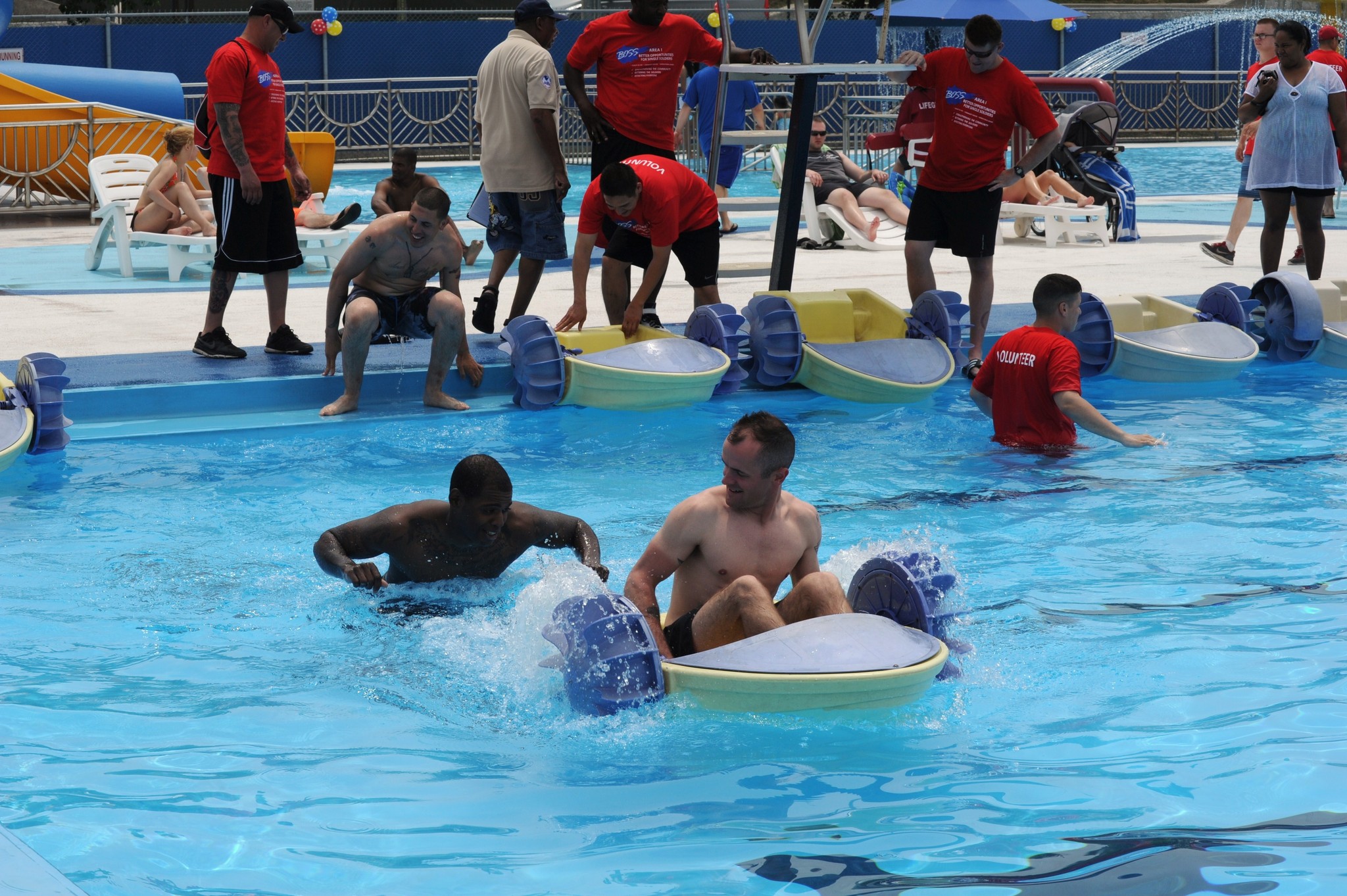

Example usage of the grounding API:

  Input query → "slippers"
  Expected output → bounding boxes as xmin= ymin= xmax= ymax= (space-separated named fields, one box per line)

xmin=330 ymin=203 xmax=362 ymax=231
xmin=719 ymin=223 xmax=738 ymax=237
xmin=796 ymin=237 xmax=844 ymax=250
xmin=962 ymin=359 xmax=984 ymax=380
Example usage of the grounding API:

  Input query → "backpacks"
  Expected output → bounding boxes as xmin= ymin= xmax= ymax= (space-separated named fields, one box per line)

xmin=194 ymin=40 xmax=250 ymax=160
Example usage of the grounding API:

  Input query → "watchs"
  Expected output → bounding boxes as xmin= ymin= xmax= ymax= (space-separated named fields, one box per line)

xmin=1250 ymin=98 xmax=1262 ymax=108
xmin=1014 ymin=165 xmax=1025 ymax=179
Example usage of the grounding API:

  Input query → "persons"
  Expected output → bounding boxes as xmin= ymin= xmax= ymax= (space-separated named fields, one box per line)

xmin=622 ymin=410 xmax=856 ymax=662
xmin=313 ymin=453 xmax=610 ymax=594
xmin=129 ymin=0 xmax=1347 ymax=455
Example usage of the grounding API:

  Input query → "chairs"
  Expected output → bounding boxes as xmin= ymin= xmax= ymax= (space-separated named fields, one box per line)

xmin=85 ymin=154 xmax=217 ymax=282
xmin=295 ymin=192 xmax=348 ymax=268
xmin=770 ymin=144 xmax=907 ymax=251
xmin=995 ymin=172 xmax=1109 ymax=248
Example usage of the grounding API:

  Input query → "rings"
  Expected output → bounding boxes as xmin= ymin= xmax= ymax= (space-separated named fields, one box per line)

xmin=883 ymin=174 xmax=886 ymax=175
xmin=994 ymin=180 xmax=996 ymax=184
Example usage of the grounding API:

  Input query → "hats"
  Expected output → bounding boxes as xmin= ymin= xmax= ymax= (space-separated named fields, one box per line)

xmin=249 ymin=0 xmax=305 ymax=34
xmin=1318 ymin=26 xmax=1343 ymax=40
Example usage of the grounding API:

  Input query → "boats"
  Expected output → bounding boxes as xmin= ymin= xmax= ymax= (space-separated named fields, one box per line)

xmin=1245 ymin=270 xmax=1347 ymax=373
xmin=534 ymin=541 xmax=971 ymax=717
xmin=1055 ymin=281 xmax=1267 ymax=384
xmin=739 ymin=286 xmax=972 ymax=407
xmin=0 ymin=352 xmax=74 ymax=474
xmin=498 ymin=303 xmax=753 ymax=414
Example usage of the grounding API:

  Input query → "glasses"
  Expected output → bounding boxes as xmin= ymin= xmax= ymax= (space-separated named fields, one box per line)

xmin=272 ymin=18 xmax=288 ymax=35
xmin=1251 ymin=33 xmax=1274 ymax=39
xmin=963 ymin=39 xmax=995 ymax=59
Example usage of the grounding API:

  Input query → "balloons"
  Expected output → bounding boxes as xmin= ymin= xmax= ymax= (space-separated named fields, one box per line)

xmin=1064 ymin=18 xmax=1076 ymax=21
xmin=713 ymin=1 xmax=729 ymax=14
xmin=728 ymin=13 xmax=734 ymax=25
xmin=707 ymin=13 xmax=721 ymax=27
xmin=1065 ymin=23 xmax=1078 ymax=32
xmin=1051 ymin=18 xmax=1065 ymax=30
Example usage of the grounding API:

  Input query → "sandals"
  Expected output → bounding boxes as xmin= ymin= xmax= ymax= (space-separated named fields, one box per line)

xmin=500 ymin=319 xmax=512 ymax=340
xmin=472 ymin=286 xmax=499 ymax=334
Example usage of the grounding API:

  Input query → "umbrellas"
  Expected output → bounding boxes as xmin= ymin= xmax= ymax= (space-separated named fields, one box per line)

xmin=868 ymin=0 xmax=1089 ymax=29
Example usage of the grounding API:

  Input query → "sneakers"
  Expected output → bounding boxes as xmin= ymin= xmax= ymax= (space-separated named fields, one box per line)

xmin=1287 ymin=245 xmax=1306 ymax=265
xmin=1199 ymin=241 xmax=1236 ymax=265
xmin=640 ymin=302 xmax=671 ymax=334
xmin=264 ymin=324 xmax=314 ymax=354
xmin=192 ymin=325 xmax=248 ymax=359
